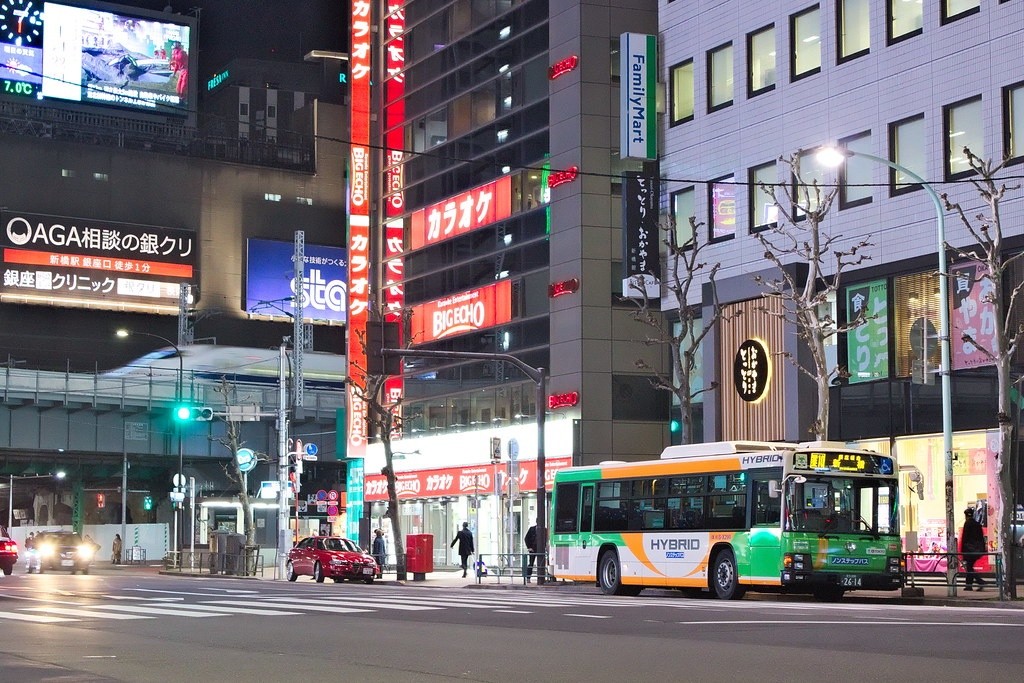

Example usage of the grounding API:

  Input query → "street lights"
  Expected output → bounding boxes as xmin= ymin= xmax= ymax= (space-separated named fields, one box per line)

xmin=8 ymin=472 xmax=66 ymax=540
xmin=117 ymin=330 xmax=183 ymax=567
xmin=816 ymin=144 xmax=958 ymax=598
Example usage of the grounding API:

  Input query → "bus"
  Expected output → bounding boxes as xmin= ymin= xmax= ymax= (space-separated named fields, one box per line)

xmin=550 ymin=441 xmax=924 ymax=602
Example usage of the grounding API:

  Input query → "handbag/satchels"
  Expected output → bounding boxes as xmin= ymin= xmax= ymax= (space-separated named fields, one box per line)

xmin=91 ymin=543 xmax=101 ymax=553
xmin=376 ymin=564 xmax=380 ymax=574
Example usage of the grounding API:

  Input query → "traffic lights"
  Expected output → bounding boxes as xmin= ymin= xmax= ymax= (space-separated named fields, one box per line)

xmin=144 ymin=496 xmax=152 ymax=510
xmin=174 ymin=406 xmax=213 ymax=422
xmin=98 ymin=494 xmax=105 ymax=508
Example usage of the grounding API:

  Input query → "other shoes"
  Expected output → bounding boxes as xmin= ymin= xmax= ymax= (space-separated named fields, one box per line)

xmin=977 ymin=580 xmax=986 ymax=591
xmin=964 ymin=587 xmax=974 ymax=591
xmin=527 ymin=579 xmax=531 ymax=583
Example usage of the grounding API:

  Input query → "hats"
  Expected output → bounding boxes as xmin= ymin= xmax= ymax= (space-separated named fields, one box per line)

xmin=964 ymin=508 xmax=975 ymax=517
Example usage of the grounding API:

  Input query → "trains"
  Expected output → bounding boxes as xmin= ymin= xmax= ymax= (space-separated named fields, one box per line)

xmin=103 ymin=344 xmax=346 ymax=388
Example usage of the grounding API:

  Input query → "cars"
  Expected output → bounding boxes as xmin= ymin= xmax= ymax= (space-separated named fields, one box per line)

xmin=26 ymin=530 xmax=89 ymax=575
xmin=284 ymin=536 xmax=378 ymax=584
xmin=0 ymin=525 xmax=18 ymax=575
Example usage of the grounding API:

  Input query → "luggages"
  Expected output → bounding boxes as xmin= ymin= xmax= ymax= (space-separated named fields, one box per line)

xmin=470 ymin=554 xmax=488 ymax=578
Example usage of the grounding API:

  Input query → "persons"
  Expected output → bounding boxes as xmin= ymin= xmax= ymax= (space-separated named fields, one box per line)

xmin=372 ymin=529 xmax=387 ymax=579
xmin=960 ymin=508 xmax=986 ymax=590
xmin=450 ymin=522 xmax=475 ymax=578
xmin=25 ymin=530 xmax=40 ymax=547
xmin=152 ymin=41 xmax=189 ymax=98
xmin=112 ymin=534 xmax=122 ymax=564
xmin=525 ymin=518 xmax=547 ymax=583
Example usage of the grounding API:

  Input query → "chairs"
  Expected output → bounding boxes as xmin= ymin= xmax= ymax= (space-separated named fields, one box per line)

xmin=582 ymin=504 xmax=703 ymax=529
